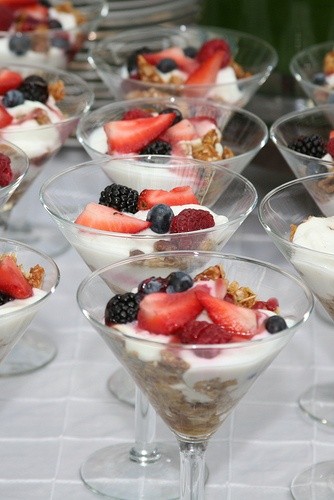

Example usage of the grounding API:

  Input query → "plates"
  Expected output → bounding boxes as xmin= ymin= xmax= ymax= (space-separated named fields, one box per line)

xmin=60 ymin=1 xmax=201 ymax=150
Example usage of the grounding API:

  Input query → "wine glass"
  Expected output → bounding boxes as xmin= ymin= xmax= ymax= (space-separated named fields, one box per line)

xmin=76 ymin=97 xmax=268 ymax=409
xmin=75 ymin=250 xmax=315 ymax=499
xmin=0 ymin=0 xmax=108 ymax=258
xmin=255 ymin=172 xmax=334 ymax=500
xmin=268 ymin=103 xmax=333 ymax=428
xmin=39 ymin=154 xmax=259 ymax=500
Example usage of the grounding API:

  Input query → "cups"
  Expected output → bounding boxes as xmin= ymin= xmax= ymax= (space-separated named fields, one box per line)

xmin=0 ymin=237 xmax=61 ymax=370
xmin=0 ymin=61 xmax=95 ymax=223
xmin=88 ymin=23 xmax=279 ymax=111
xmin=286 ymin=34 xmax=334 ymax=106
xmin=0 ymin=135 xmax=31 ymax=216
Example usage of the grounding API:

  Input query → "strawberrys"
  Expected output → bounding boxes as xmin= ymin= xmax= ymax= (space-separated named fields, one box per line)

xmin=139 ymin=284 xmax=268 ymax=340
xmin=327 ymin=131 xmax=334 ymax=158
xmin=0 ymin=6 xmax=223 ymax=300
